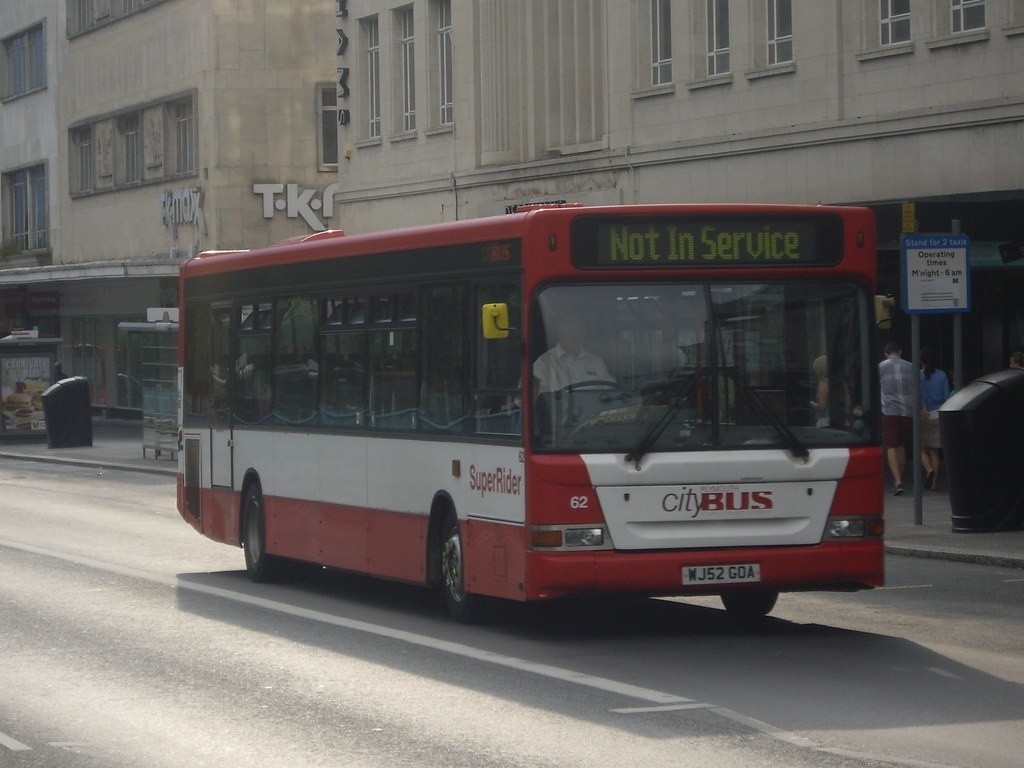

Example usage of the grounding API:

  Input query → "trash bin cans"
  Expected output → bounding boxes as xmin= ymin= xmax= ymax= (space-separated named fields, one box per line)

xmin=937 ymin=369 xmax=1024 ymax=534
xmin=40 ymin=376 xmax=92 ymax=449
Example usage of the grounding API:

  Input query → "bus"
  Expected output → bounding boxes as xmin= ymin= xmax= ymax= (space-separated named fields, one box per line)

xmin=174 ymin=202 xmax=897 ymax=623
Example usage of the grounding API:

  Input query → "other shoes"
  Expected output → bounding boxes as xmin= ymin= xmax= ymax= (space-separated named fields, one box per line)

xmin=926 ymin=471 xmax=935 ymax=488
xmin=930 ymin=484 xmax=938 ymax=490
xmin=892 ymin=484 xmax=904 ymax=496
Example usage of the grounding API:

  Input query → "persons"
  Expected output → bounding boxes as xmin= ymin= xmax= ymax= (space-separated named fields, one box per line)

xmin=809 ymin=355 xmax=850 ymax=430
xmin=1010 ymin=353 xmax=1024 ymax=369
xmin=513 ymin=317 xmax=616 ymax=407
xmin=919 ymin=348 xmax=949 ymax=491
xmin=54 ymin=362 xmax=68 ymax=384
xmin=877 ymin=342 xmax=912 ymax=495
